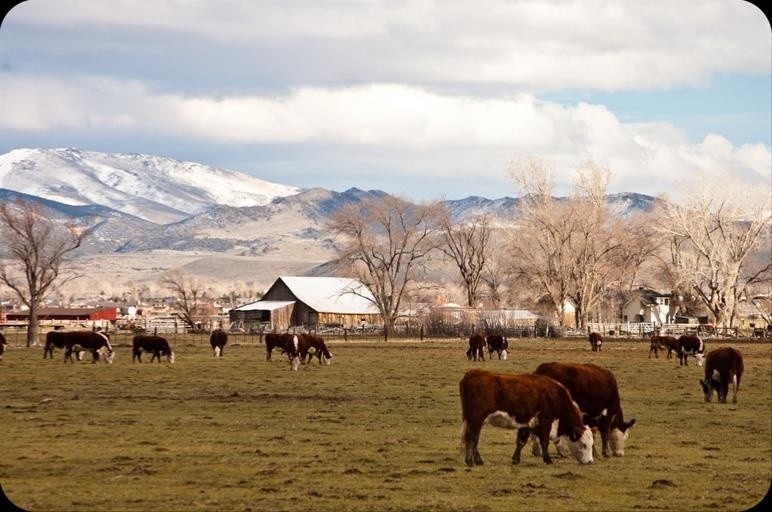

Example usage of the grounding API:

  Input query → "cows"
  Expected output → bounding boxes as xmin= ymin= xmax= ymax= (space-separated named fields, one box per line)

xmin=648 ymin=336 xmax=682 ymax=359
xmin=42 ymin=331 xmax=115 ymax=365
xmin=589 ymin=332 xmax=603 ymax=352
xmin=0 ymin=332 xmax=10 ymax=362
xmin=456 ymin=368 xmax=597 ymax=464
xmin=210 ymin=329 xmax=228 ymax=357
xmin=531 ymin=361 xmax=636 ymax=458
xmin=265 ymin=333 xmax=333 ymax=371
xmin=699 ymin=347 xmax=744 ymax=411
xmin=465 ymin=335 xmax=487 ymax=362
xmin=132 ymin=335 xmax=176 ymax=366
xmin=676 ymin=336 xmax=707 ymax=367
xmin=486 ymin=335 xmax=509 ymax=362
